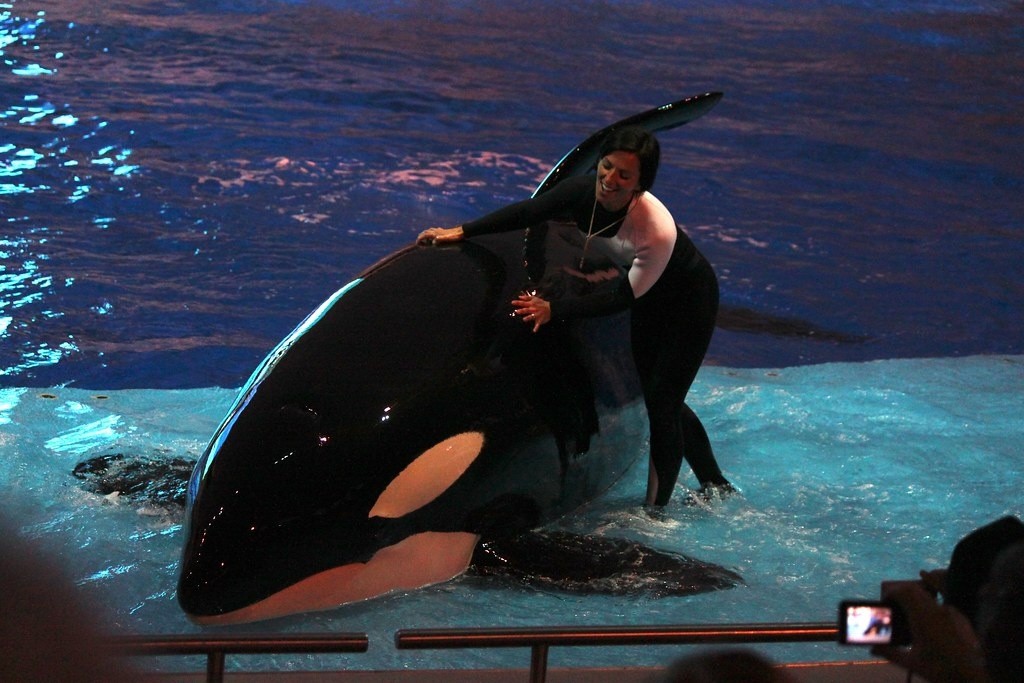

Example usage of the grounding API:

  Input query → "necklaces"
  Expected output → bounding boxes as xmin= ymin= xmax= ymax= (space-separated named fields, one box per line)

xmin=576 ymin=191 xmax=640 ymax=268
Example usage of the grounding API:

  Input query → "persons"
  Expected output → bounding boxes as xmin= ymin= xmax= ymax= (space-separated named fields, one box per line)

xmin=658 ymin=514 xmax=1023 ymax=683
xmin=416 ymin=126 xmax=736 ymax=521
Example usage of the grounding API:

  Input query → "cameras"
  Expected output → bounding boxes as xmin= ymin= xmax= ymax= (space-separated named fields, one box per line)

xmin=837 ymin=601 xmax=914 ymax=647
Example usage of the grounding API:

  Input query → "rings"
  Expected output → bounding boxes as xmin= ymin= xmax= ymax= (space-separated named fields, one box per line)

xmin=531 ymin=314 xmax=535 ymax=319
